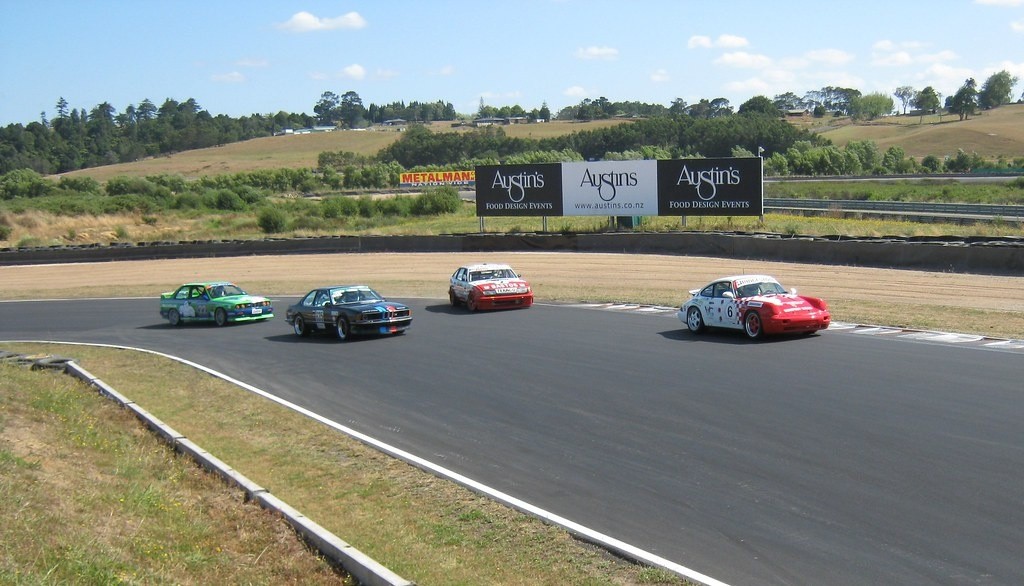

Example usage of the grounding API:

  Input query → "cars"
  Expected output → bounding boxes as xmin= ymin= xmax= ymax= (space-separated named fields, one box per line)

xmin=284 ymin=285 xmax=412 ymax=343
xmin=449 ymin=263 xmax=533 ymax=312
xmin=160 ymin=281 xmax=274 ymax=326
xmin=678 ymin=274 xmax=832 ymax=343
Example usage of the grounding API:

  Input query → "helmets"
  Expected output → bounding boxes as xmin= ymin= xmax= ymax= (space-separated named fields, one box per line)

xmin=216 ymin=287 xmax=225 ymax=294
